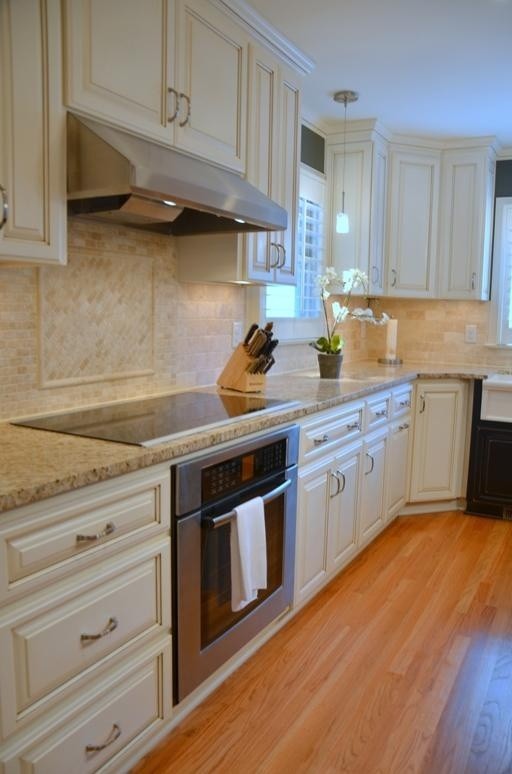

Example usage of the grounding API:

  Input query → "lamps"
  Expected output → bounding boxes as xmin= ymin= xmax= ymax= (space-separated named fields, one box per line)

xmin=332 ymin=90 xmax=358 ymax=235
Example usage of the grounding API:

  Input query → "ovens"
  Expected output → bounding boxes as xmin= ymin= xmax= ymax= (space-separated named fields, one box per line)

xmin=170 ymin=424 xmax=301 ymax=709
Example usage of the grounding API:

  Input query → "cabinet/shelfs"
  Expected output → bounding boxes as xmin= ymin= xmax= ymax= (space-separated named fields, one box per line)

xmin=66 ymin=1 xmax=249 ymax=180
xmin=385 ymin=134 xmax=437 ymax=300
xmin=317 ymin=118 xmax=385 ymax=298
xmin=309 ymin=266 xmax=390 ymax=356
xmin=0 ymin=462 xmax=175 ymax=774
xmin=174 ymin=0 xmax=315 ymax=287
xmin=437 ymin=136 xmax=497 ymax=302
xmin=1 ymin=0 xmax=67 ymax=268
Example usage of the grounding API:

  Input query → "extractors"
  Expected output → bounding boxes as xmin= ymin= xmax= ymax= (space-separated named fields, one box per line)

xmin=66 ymin=112 xmax=288 ymax=237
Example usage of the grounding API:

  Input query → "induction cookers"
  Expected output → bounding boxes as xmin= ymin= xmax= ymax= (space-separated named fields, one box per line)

xmin=10 ymin=391 xmax=303 ymax=450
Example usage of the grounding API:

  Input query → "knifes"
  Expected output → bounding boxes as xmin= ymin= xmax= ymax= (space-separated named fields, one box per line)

xmin=242 ymin=320 xmax=279 ymax=375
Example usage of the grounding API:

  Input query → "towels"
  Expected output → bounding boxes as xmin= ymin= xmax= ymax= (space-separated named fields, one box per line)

xmin=229 ymin=496 xmax=268 ymax=612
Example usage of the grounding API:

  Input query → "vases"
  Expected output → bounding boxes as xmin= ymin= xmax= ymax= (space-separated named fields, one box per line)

xmin=317 ymin=354 xmax=343 ymax=380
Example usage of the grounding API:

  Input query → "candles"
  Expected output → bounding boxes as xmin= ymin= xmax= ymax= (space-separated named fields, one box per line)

xmin=385 ymin=315 xmax=397 ymax=360
